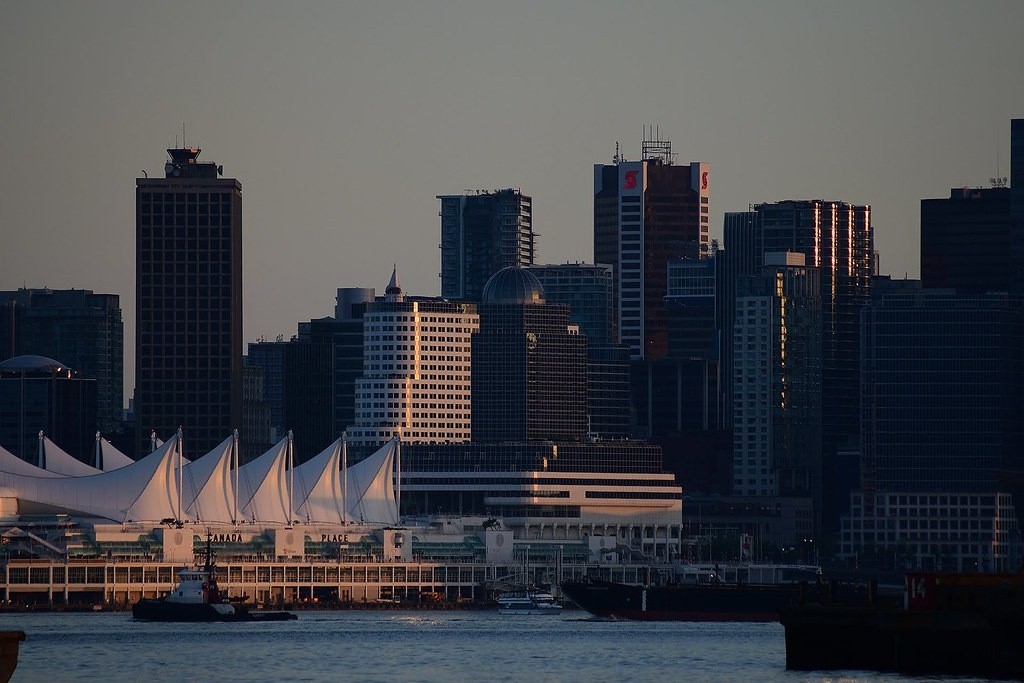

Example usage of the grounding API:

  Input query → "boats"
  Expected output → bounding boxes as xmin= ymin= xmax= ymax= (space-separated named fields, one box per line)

xmin=498 ymin=587 xmax=562 ymax=615
xmin=132 ymin=528 xmax=298 ymax=622
xmin=561 ymin=571 xmax=879 ymax=622
